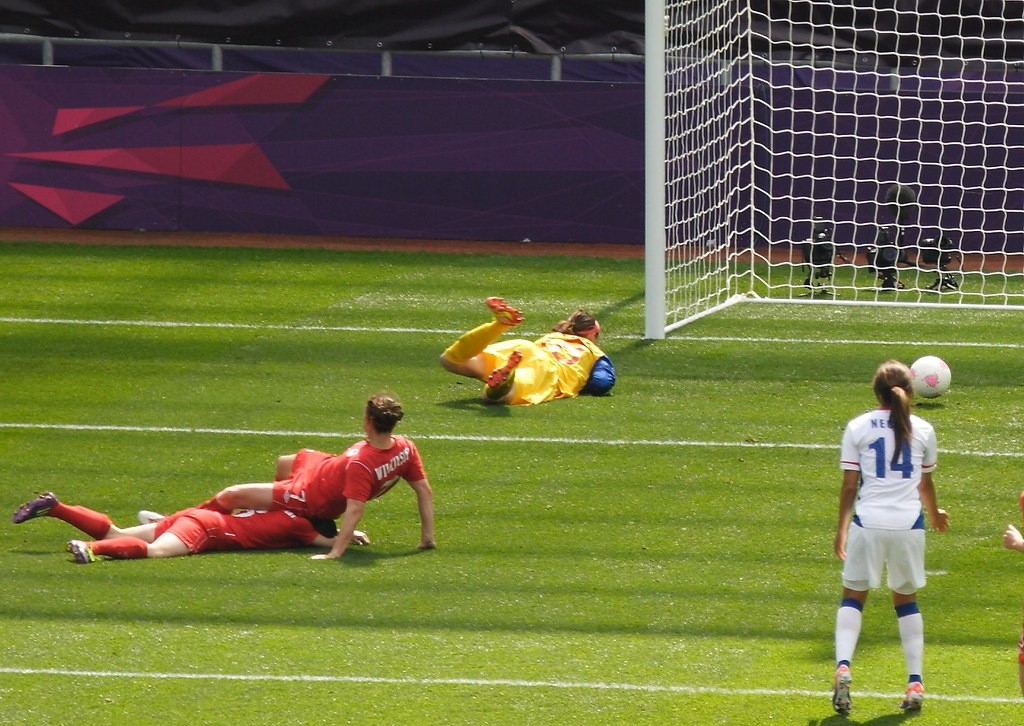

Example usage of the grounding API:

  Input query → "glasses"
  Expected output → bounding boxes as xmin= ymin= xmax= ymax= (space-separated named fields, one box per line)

xmin=594 ymin=333 xmax=600 ymax=338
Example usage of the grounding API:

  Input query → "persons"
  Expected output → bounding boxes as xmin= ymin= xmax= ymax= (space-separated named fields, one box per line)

xmin=15 ymin=490 xmax=371 ymax=566
xmin=440 ymin=295 xmax=618 ymax=406
xmin=138 ymin=394 xmax=437 ymax=560
xmin=1002 ymin=491 xmax=1024 ymax=699
xmin=830 ymin=358 xmax=952 ymax=713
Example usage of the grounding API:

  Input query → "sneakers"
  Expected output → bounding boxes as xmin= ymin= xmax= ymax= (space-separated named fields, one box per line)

xmin=13 ymin=491 xmax=57 ymax=524
xmin=486 ymin=297 xmax=524 ymax=326
xmin=900 ymin=682 xmax=923 ymax=711
xmin=67 ymin=540 xmax=113 ymax=564
xmin=487 ymin=351 xmax=523 ymax=388
xmin=829 ymin=665 xmax=851 ymax=715
xmin=137 ymin=510 xmax=165 ymax=524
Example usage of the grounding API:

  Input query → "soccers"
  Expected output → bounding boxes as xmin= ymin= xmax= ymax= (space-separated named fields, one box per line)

xmin=908 ymin=356 xmax=952 ymax=400
